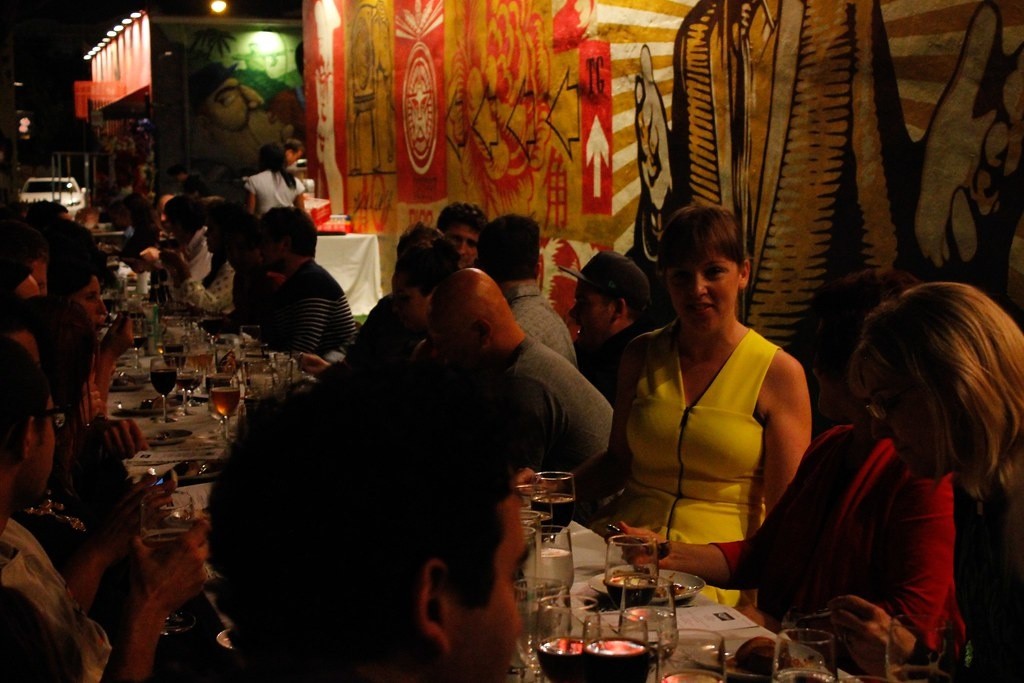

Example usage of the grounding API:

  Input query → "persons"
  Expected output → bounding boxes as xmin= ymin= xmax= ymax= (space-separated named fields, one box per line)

xmin=607 ymin=264 xmax=964 ymax=682
xmin=510 ymin=205 xmax=813 ymax=631
xmin=850 ymin=281 xmax=1024 ymax=683
xmin=0 ymin=140 xmax=668 ymax=683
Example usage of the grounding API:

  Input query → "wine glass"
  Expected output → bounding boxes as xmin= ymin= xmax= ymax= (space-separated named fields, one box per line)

xmin=132 ymin=316 xmax=304 ymax=447
xmin=139 ymin=488 xmax=197 ymax=636
xmin=514 ymin=471 xmax=725 ymax=683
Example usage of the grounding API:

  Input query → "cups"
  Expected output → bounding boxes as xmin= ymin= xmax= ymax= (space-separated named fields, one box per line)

xmin=773 ymin=626 xmax=841 ymax=683
xmin=883 ymin=614 xmax=961 ymax=682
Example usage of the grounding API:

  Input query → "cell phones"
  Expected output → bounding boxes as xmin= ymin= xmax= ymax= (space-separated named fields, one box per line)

xmin=154 ymin=473 xmax=172 ymax=485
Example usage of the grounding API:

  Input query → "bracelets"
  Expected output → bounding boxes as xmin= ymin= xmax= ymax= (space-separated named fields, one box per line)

xmin=151 ymin=260 xmax=161 ymax=266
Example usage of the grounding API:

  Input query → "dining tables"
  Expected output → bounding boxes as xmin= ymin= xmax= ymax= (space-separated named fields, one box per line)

xmin=101 ymin=236 xmax=865 ymax=683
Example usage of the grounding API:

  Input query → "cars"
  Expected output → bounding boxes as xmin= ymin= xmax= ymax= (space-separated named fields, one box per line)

xmin=18 ymin=178 xmax=86 ymax=218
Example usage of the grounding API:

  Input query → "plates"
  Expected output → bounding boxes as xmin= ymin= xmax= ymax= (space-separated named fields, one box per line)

xmin=582 ymin=566 xmax=703 ymax=601
xmin=694 ymin=636 xmax=825 ymax=682
xmin=216 ymin=627 xmax=232 ymax=649
xmin=110 ymin=353 xmax=280 ymax=482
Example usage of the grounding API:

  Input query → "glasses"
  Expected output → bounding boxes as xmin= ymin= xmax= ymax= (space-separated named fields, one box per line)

xmin=865 ymin=380 xmax=922 ymax=419
xmin=26 ymin=401 xmax=71 ymax=428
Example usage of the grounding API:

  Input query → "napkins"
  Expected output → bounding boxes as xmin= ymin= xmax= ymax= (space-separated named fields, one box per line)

xmin=550 ymin=521 xmax=627 ymax=570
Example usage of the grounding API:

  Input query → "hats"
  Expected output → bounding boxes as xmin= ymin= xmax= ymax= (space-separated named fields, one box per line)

xmin=559 ymin=249 xmax=648 ymax=307
